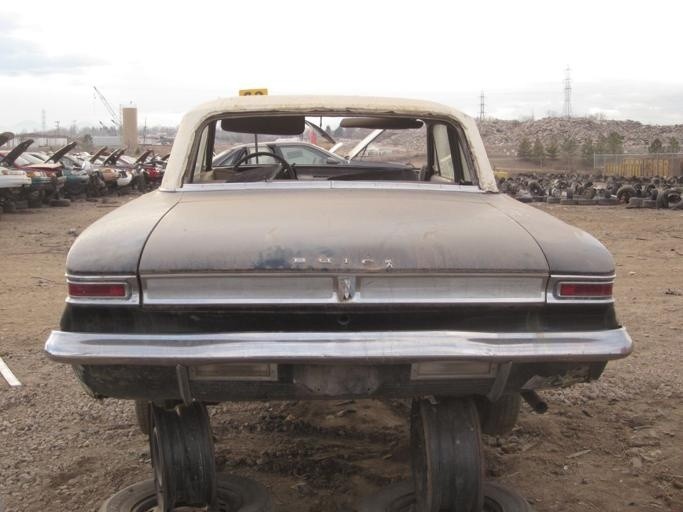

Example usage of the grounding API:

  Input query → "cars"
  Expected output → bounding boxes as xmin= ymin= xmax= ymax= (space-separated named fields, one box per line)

xmin=44 ymin=92 xmax=635 ymax=510
xmin=1 ymin=128 xmax=170 ymax=209
